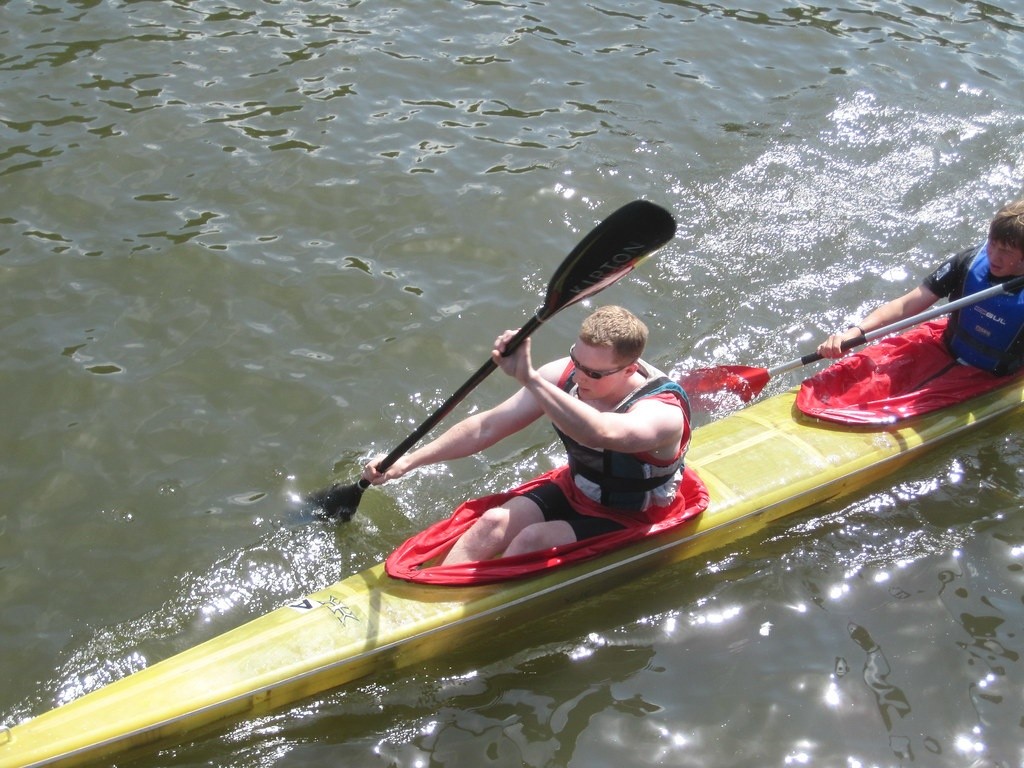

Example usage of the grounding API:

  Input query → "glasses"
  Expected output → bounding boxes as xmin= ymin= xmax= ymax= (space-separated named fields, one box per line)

xmin=569 ymin=344 xmax=635 ymax=379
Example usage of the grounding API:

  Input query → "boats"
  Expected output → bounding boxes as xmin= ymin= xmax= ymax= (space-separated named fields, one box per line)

xmin=0 ymin=310 xmax=1022 ymax=768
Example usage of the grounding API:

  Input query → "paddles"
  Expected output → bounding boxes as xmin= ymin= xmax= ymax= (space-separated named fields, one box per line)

xmin=301 ymin=196 xmax=691 ymax=533
xmin=662 ymin=266 xmax=1024 ymax=423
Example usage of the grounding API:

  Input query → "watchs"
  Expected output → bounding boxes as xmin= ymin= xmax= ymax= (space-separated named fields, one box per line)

xmin=848 ymin=323 xmax=865 ymax=336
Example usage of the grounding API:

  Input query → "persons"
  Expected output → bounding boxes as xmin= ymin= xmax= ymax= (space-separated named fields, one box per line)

xmin=817 ymin=200 xmax=1024 ymax=377
xmin=362 ymin=305 xmax=692 ymax=565
